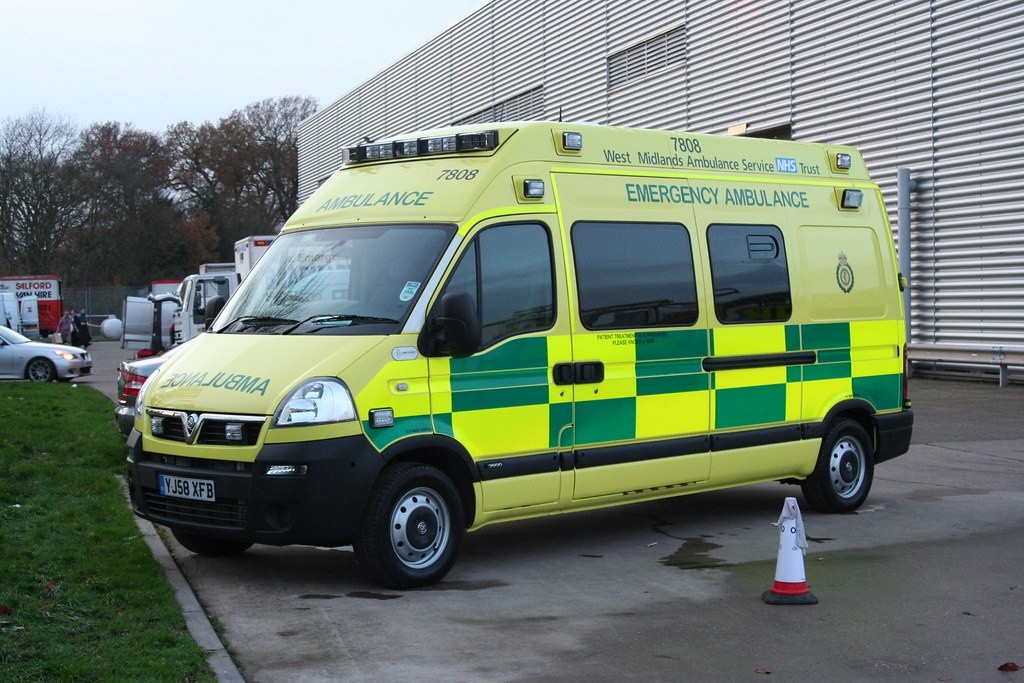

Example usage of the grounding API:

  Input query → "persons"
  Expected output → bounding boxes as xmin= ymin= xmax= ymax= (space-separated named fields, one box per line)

xmin=68 ymin=309 xmax=93 ymax=350
xmin=56 ymin=310 xmax=79 ymax=346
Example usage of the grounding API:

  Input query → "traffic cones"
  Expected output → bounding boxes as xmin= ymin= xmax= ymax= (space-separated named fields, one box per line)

xmin=759 ymin=496 xmax=821 ymax=607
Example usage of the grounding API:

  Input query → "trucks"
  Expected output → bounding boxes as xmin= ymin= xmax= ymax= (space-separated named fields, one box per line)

xmin=170 ymin=234 xmax=350 ymax=348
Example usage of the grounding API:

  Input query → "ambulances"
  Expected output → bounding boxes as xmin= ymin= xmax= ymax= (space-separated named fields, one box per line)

xmin=124 ymin=120 xmax=918 ymax=589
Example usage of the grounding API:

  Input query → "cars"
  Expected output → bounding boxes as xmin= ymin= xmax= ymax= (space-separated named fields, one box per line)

xmin=0 ymin=325 xmax=94 ymax=385
xmin=112 ymin=336 xmax=198 ymax=439
